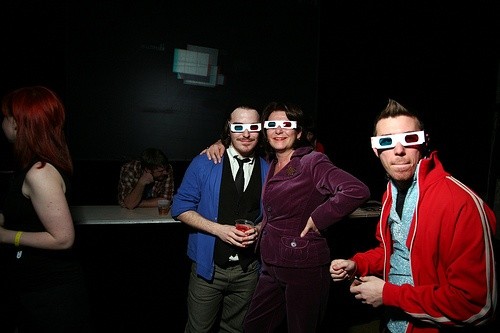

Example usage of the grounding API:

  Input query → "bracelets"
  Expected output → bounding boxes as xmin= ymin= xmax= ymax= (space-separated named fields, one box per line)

xmin=15 ymin=232 xmax=22 ymax=247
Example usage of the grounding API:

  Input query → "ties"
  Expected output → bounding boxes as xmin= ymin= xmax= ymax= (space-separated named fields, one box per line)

xmin=231 ymin=155 xmax=251 ymax=257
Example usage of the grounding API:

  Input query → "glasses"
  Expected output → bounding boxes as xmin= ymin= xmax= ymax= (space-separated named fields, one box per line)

xmin=264 ymin=121 xmax=297 ymax=129
xmin=370 ymin=131 xmax=425 ymax=149
xmin=228 ymin=121 xmax=261 ymax=132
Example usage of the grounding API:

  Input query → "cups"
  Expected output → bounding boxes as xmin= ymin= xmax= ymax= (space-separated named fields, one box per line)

xmin=157 ymin=199 xmax=170 ymax=216
xmin=235 ymin=219 xmax=253 ymax=242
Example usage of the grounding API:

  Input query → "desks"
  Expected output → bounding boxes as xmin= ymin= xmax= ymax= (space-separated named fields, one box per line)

xmin=69 ymin=206 xmax=193 ymax=224
xmin=347 ymin=202 xmax=382 ymax=218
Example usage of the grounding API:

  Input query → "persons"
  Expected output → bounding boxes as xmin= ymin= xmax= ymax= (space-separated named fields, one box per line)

xmin=330 ymin=99 xmax=500 ymax=333
xmin=118 ymin=148 xmax=175 ymax=209
xmin=198 ymin=102 xmax=371 ymax=333
xmin=171 ymin=101 xmax=271 ymax=333
xmin=0 ymin=86 xmax=94 ymax=333
